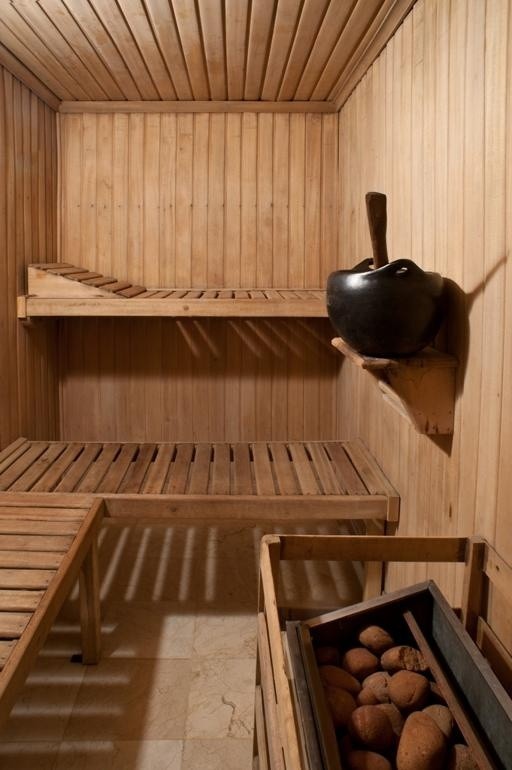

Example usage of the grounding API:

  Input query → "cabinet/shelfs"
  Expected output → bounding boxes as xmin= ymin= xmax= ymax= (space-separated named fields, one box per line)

xmin=2 ymin=491 xmax=106 ymax=722
xmin=2 ymin=434 xmax=401 ymax=601
